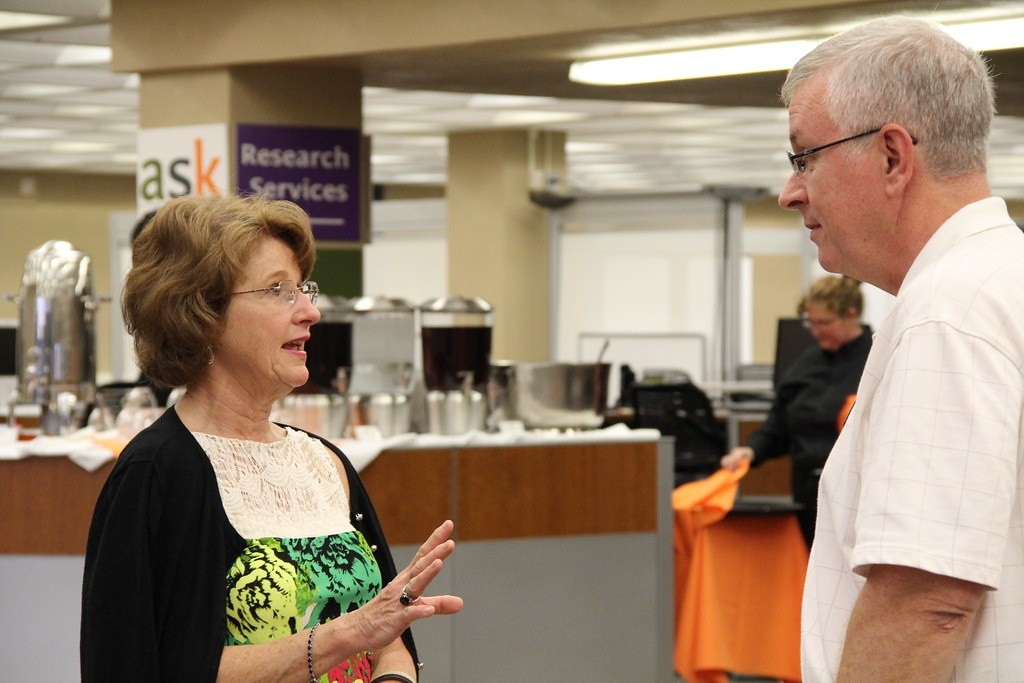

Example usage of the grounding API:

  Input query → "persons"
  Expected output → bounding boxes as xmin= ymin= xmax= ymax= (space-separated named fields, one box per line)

xmin=80 ymin=197 xmax=464 ymax=683
xmin=721 ymin=274 xmax=875 ymax=554
xmin=778 ymin=17 xmax=1024 ymax=683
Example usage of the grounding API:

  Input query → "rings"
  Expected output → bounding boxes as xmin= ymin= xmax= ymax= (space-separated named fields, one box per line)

xmin=399 ymin=584 xmax=421 ymax=606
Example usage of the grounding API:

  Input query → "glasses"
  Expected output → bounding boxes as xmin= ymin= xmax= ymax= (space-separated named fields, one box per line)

xmin=802 ymin=317 xmax=838 ymax=329
xmin=210 ymin=279 xmax=319 ymax=307
xmin=786 ymin=127 xmax=921 ymax=174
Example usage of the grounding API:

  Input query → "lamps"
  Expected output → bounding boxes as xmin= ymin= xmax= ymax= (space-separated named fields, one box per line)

xmin=568 ymin=0 xmax=1024 ymax=86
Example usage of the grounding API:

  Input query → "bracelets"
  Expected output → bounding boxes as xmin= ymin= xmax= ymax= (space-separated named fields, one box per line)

xmin=370 ymin=670 xmax=414 ymax=683
xmin=307 ymin=622 xmax=320 ymax=683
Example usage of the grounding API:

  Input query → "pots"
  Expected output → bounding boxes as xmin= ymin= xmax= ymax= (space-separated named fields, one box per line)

xmin=489 ymin=359 xmax=611 ymax=428
